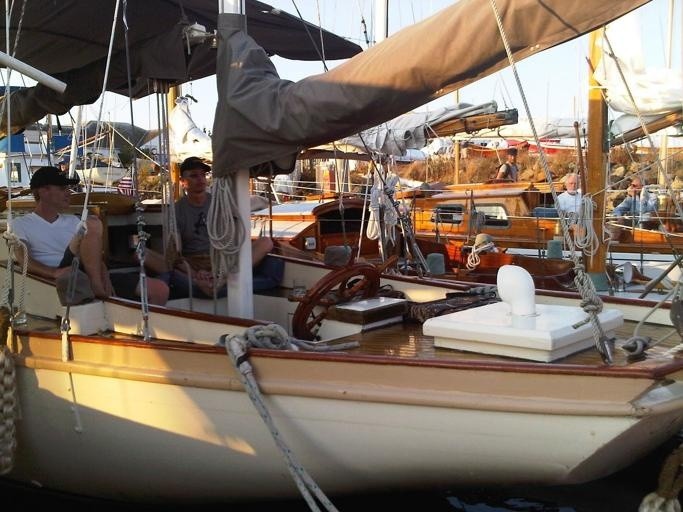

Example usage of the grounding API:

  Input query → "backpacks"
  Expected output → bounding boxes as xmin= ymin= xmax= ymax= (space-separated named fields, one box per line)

xmin=488 ymin=162 xmax=510 ymax=181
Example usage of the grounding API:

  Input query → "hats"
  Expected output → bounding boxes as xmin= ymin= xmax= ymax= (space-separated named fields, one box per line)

xmin=507 ymin=149 xmax=518 ymax=156
xmin=178 ymin=157 xmax=212 ymax=176
xmin=28 ymin=165 xmax=81 ymax=187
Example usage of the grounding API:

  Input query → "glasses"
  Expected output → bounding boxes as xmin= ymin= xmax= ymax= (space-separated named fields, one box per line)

xmin=630 ymin=183 xmax=642 ymax=188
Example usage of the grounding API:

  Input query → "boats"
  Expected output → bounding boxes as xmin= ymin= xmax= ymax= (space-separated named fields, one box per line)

xmin=507 ymin=139 xmax=575 ymax=155
xmin=463 ymin=139 xmax=530 ymax=161
xmin=1 ymin=105 xmax=164 ymax=197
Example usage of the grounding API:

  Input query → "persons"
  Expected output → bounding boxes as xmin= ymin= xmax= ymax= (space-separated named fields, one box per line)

xmin=612 ymin=177 xmax=658 ymax=224
xmin=135 ymin=157 xmax=273 ymax=299
xmin=495 ymin=148 xmax=518 ymax=183
xmin=11 ymin=166 xmax=168 ymax=306
xmin=556 ymin=173 xmax=581 ymax=214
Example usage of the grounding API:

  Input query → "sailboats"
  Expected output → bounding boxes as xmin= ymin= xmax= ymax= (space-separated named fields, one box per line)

xmin=393 ymin=87 xmax=682 ymax=256
xmin=0 ymin=0 xmax=682 ymax=497
xmin=168 ymin=2 xmax=683 ymax=301
